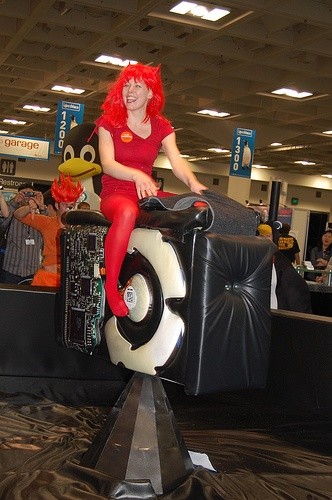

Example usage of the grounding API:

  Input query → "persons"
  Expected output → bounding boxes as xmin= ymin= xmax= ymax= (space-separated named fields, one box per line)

xmin=94 ymin=63 xmax=210 ymax=317
xmin=255 ymin=220 xmax=332 ymax=314
xmin=0 ymin=172 xmax=91 ymax=287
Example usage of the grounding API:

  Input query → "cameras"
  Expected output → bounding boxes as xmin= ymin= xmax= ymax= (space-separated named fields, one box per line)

xmin=24 ymin=192 xmax=36 ymax=198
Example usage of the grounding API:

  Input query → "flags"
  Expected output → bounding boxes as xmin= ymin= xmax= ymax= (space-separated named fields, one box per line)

xmin=54 ymin=100 xmax=84 ymax=156
xmin=229 ymin=128 xmax=256 ymax=179
